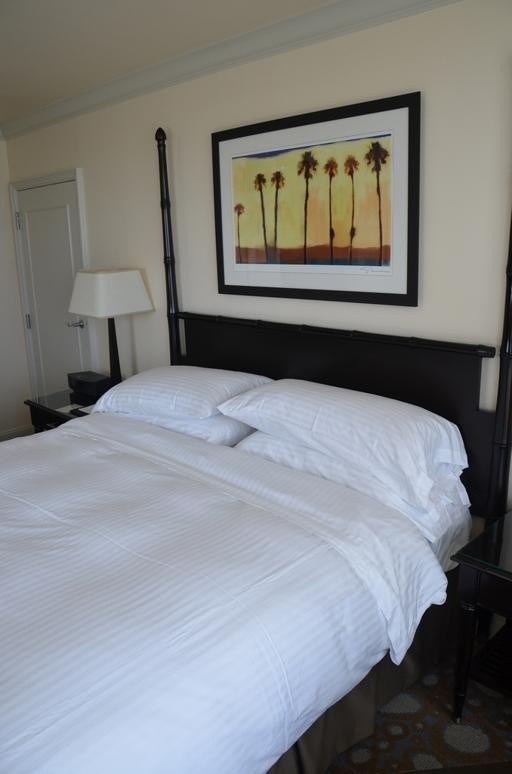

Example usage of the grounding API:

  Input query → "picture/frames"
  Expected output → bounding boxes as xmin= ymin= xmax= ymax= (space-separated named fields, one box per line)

xmin=209 ymin=89 xmax=420 ymax=307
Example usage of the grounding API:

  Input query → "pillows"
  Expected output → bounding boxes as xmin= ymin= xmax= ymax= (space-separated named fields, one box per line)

xmin=219 ymin=378 xmax=468 ymax=503
xmin=90 ymin=365 xmax=274 ymax=415
xmin=134 ymin=410 xmax=250 ymax=448
xmin=234 ymin=429 xmax=468 ymax=543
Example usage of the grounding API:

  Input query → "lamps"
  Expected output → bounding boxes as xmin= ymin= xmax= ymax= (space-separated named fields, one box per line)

xmin=66 ymin=268 xmax=155 ymax=387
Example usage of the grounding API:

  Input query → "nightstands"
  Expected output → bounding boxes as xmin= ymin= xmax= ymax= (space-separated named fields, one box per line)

xmin=22 ymin=389 xmax=97 ymax=434
xmin=451 ymin=507 xmax=511 ymax=724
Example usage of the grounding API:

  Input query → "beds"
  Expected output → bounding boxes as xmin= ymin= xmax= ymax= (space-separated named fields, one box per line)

xmin=0 ymin=311 xmax=508 ymax=774
